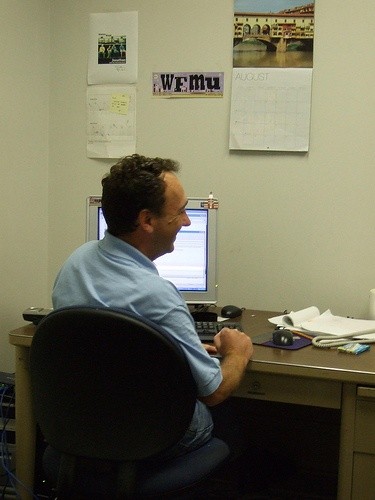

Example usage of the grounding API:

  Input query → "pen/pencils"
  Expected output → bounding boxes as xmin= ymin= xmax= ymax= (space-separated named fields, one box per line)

xmin=291 ymin=330 xmax=313 ymax=340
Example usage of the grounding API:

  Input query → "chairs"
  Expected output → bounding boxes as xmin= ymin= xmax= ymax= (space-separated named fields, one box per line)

xmin=27 ymin=304 xmax=230 ymax=500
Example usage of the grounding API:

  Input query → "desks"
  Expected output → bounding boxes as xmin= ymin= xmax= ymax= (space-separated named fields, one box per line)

xmin=8 ymin=306 xmax=375 ymax=500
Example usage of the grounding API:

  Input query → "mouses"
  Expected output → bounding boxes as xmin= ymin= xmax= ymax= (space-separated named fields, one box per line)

xmin=221 ymin=305 xmax=242 ymax=318
xmin=274 ymin=330 xmax=293 ymax=346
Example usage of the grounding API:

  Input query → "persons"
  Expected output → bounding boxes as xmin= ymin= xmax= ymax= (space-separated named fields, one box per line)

xmin=99 ymin=43 xmax=126 ymax=58
xmin=52 ymin=154 xmax=271 ymax=500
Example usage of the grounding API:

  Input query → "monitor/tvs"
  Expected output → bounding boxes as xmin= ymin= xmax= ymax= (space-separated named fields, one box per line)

xmin=85 ymin=195 xmax=217 ymax=302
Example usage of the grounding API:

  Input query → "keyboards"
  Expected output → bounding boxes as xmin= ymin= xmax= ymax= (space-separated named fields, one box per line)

xmin=193 ymin=321 xmax=241 ymax=342
